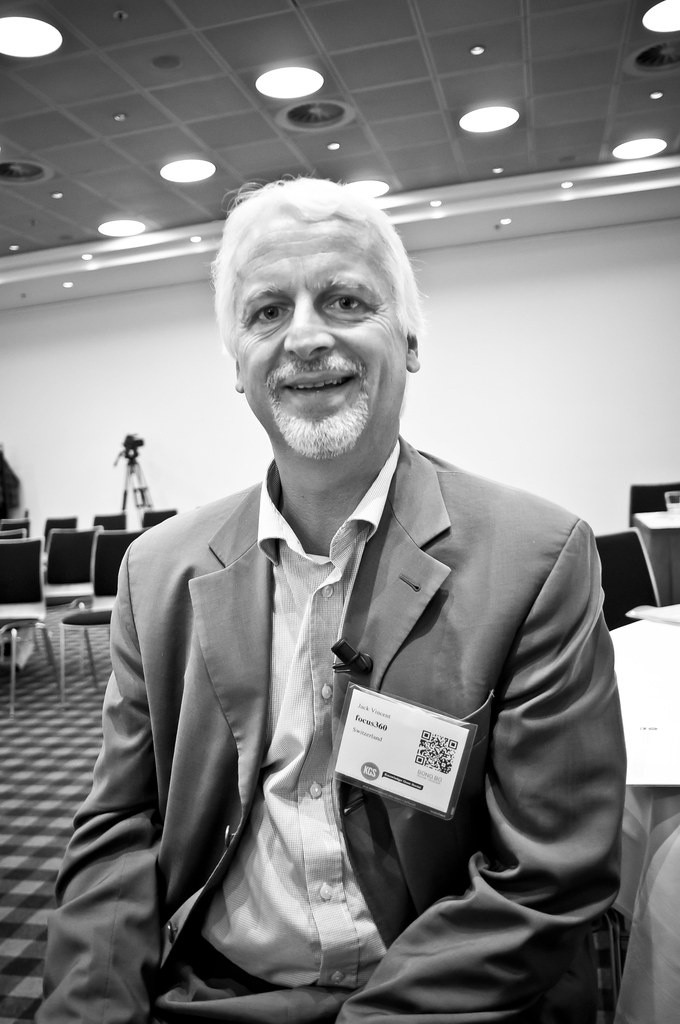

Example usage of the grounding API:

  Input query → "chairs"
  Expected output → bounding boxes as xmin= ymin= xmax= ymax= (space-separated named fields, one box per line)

xmin=594 ymin=529 xmax=658 ymax=630
xmin=0 ymin=509 xmax=178 ymax=719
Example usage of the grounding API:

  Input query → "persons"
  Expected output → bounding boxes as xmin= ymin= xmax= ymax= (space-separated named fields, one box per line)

xmin=38 ymin=179 xmax=626 ymax=1024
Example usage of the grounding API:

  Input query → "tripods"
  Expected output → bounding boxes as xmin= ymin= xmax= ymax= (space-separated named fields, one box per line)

xmin=114 ymin=448 xmax=156 ymax=527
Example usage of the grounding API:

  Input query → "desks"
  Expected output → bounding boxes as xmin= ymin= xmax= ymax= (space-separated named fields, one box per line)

xmin=610 ymin=603 xmax=680 ymax=787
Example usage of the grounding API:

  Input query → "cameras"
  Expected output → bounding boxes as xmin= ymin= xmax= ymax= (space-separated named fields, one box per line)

xmin=124 ymin=436 xmax=144 ymax=449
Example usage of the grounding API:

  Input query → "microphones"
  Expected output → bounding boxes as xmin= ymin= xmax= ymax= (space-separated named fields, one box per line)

xmin=331 ymin=639 xmax=374 ymax=675
